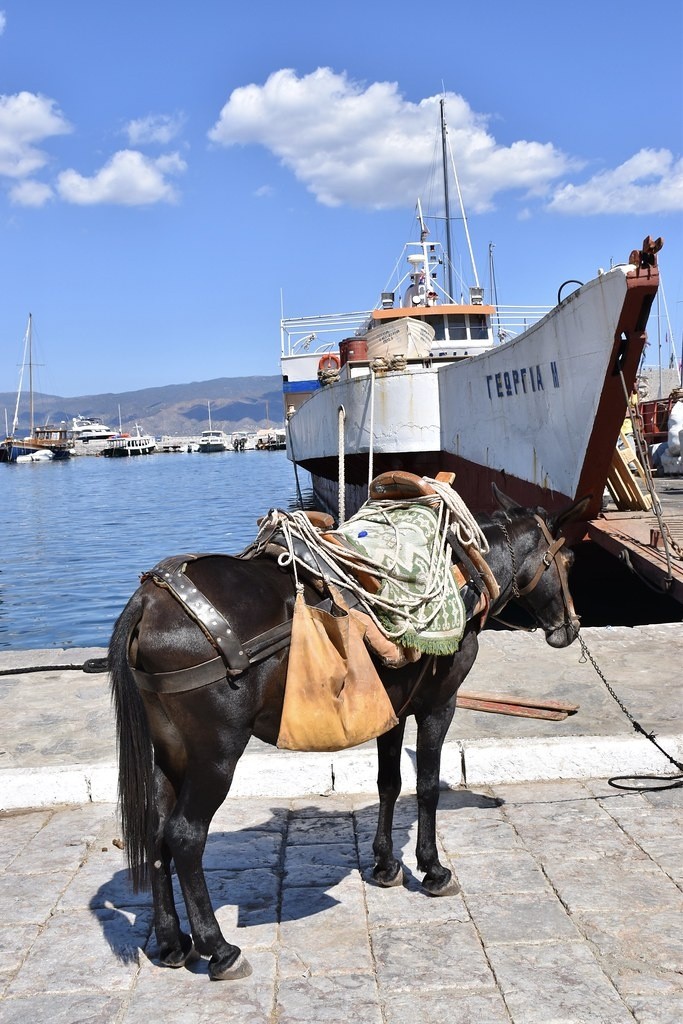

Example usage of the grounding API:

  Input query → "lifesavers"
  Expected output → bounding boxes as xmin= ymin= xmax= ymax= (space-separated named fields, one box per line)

xmin=240 ymin=439 xmax=244 ymax=446
xmin=318 ymin=354 xmax=340 ymax=371
xmin=234 ymin=439 xmax=238 ymax=447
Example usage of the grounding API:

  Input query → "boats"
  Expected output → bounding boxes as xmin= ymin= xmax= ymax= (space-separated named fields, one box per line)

xmin=279 ymin=82 xmax=667 ymax=551
xmin=54 ymin=398 xmax=287 ymax=457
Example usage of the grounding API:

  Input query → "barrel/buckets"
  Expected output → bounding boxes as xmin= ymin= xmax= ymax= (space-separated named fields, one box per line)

xmin=339 ymin=337 xmax=368 ymax=368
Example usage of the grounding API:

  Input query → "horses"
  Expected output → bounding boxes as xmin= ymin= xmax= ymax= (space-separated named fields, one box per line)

xmin=104 ymin=483 xmax=593 ymax=982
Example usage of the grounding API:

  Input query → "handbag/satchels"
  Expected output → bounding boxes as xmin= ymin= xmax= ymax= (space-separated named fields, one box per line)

xmin=276 ymin=583 xmax=400 ymax=753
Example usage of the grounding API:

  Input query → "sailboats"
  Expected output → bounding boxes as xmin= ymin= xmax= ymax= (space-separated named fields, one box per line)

xmin=0 ymin=312 xmax=76 ymax=464
